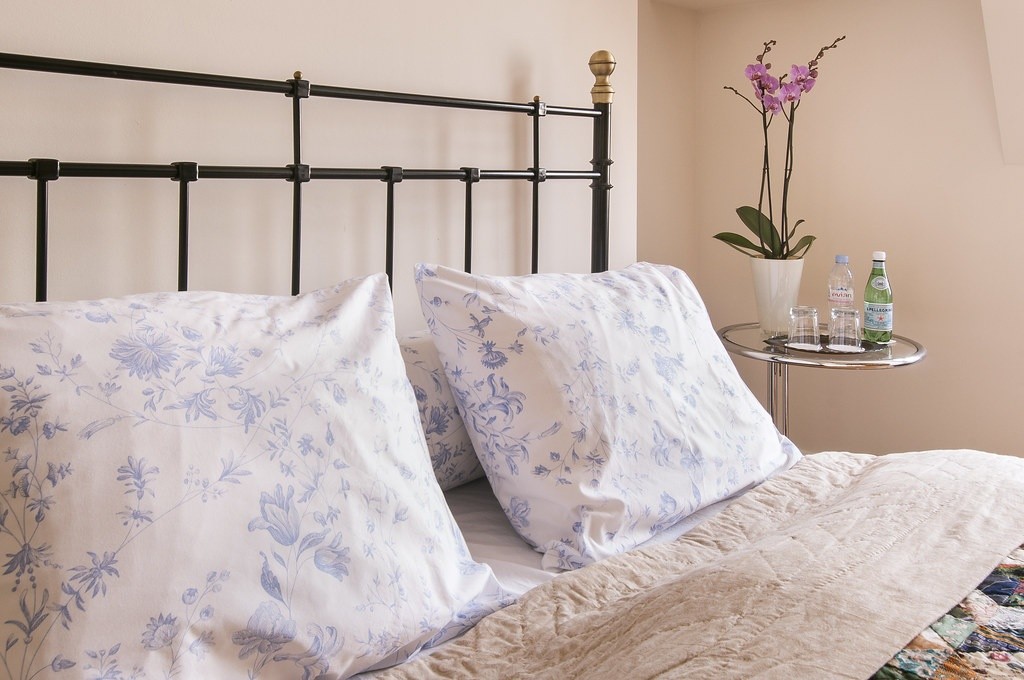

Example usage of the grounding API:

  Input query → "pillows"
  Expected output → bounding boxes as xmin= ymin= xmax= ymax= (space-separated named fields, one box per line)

xmin=0 ymin=271 xmax=523 ymax=680
xmin=398 ymin=335 xmax=483 ymax=491
xmin=409 ymin=260 xmax=801 ymax=571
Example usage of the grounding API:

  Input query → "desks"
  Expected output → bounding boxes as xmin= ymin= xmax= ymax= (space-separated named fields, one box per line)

xmin=718 ymin=322 xmax=928 ymax=438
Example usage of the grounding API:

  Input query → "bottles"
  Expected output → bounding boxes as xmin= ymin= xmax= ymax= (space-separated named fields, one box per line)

xmin=825 ymin=254 xmax=855 ymax=324
xmin=864 ymin=251 xmax=893 ymax=342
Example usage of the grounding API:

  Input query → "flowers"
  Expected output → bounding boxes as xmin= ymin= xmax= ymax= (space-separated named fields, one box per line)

xmin=710 ymin=35 xmax=848 ymax=258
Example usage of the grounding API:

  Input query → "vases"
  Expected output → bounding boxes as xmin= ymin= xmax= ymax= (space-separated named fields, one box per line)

xmin=750 ymin=256 xmax=805 ymax=333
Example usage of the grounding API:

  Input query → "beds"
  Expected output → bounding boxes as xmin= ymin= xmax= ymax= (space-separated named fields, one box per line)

xmin=0 ymin=51 xmax=1024 ymax=680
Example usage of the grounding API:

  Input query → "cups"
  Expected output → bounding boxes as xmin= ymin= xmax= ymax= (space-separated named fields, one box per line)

xmin=787 ymin=306 xmax=821 ymax=349
xmin=828 ymin=308 xmax=862 ymax=350
